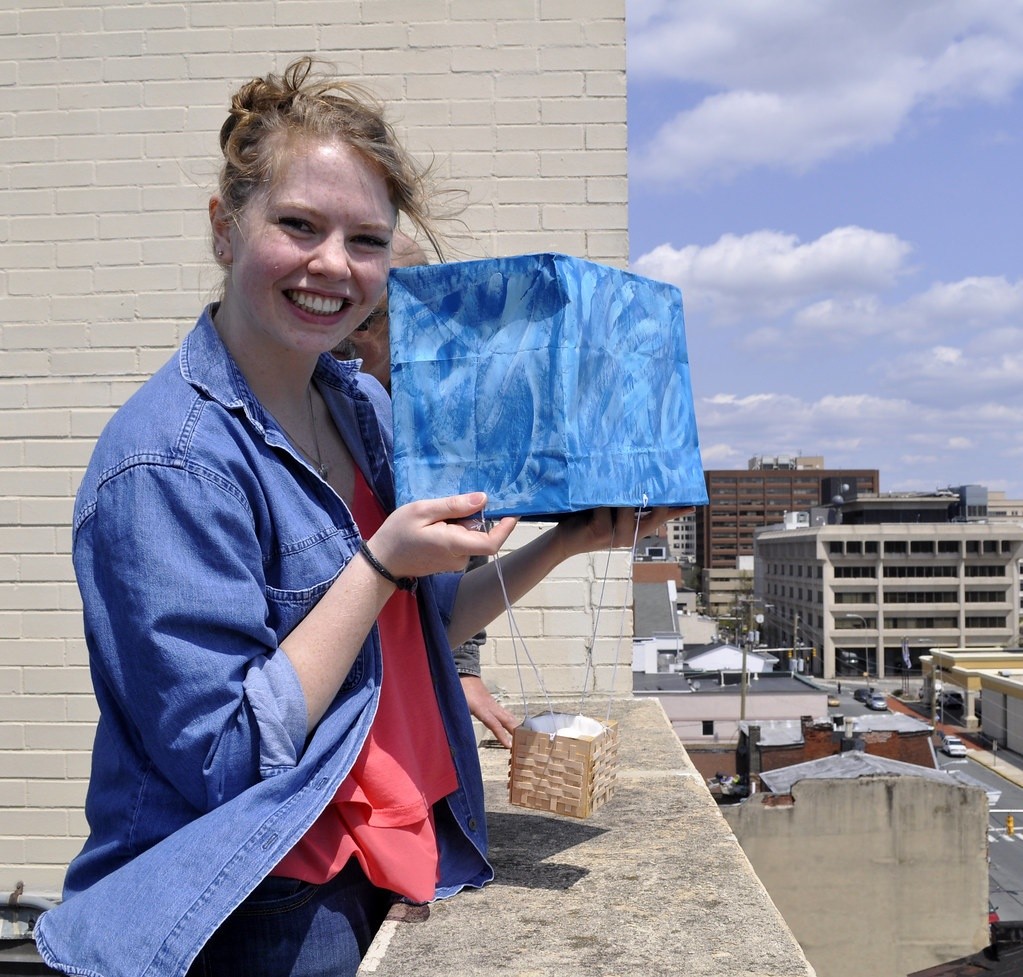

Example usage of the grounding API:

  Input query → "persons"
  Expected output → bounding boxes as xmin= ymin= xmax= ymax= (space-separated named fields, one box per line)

xmin=332 ymin=232 xmax=522 ymax=752
xmin=34 ymin=55 xmax=696 ymax=977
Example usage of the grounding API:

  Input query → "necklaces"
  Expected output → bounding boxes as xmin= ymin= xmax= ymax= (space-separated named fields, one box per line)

xmin=263 ymin=383 xmax=327 ymax=479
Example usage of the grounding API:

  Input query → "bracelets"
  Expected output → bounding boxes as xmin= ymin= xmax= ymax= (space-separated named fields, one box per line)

xmin=360 ymin=540 xmax=417 ymax=593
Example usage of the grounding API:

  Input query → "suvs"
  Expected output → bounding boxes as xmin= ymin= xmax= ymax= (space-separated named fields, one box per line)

xmin=942 ymin=735 xmax=968 ymax=758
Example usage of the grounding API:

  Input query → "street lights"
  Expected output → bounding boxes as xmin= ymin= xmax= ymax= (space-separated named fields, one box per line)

xmin=846 ymin=614 xmax=872 ymax=693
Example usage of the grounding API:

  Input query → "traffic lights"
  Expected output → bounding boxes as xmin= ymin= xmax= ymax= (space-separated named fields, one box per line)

xmin=788 ymin=651 xmax=793 ymax=658
xmin=812 ymin=649 xmax=817 ymax=657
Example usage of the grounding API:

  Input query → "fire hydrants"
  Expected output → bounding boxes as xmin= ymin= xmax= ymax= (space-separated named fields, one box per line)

xmin=1006 ymin=815 xmax=1016 ymax=836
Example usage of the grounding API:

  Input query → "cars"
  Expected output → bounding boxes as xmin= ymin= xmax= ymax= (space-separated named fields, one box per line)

xmin=828 ymin=695 xmax=840 ymax=706
xmin=854 ymin=689 xmax=873 ymax=703
xmin=866 ymin=696 xmax=888 ymax=710
